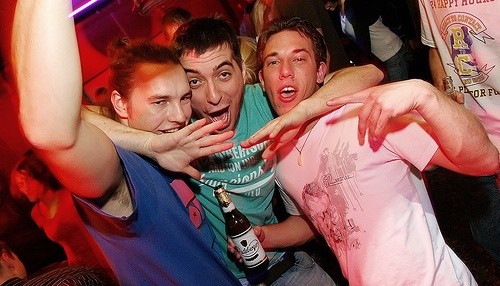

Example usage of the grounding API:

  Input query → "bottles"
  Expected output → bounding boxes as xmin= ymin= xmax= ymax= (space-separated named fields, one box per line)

xmin=441 ymin=76 xmax=457 ymax=101
xmin=213 ymin=185 xmax=270 ymax=274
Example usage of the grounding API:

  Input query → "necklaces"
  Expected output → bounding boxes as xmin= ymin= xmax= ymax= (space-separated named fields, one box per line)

xmin=295 ymin=123 xmax=315 ymax=166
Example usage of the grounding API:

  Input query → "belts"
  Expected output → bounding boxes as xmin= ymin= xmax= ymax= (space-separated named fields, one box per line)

xmin=269 ymin=254 xmax=294 ymax=285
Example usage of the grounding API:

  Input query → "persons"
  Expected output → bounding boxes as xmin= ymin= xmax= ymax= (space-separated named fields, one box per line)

xmin=0 ymin=0 xmax=500 ymax=286
xmin=227 ymin=15 xmax=500 ymax=286
